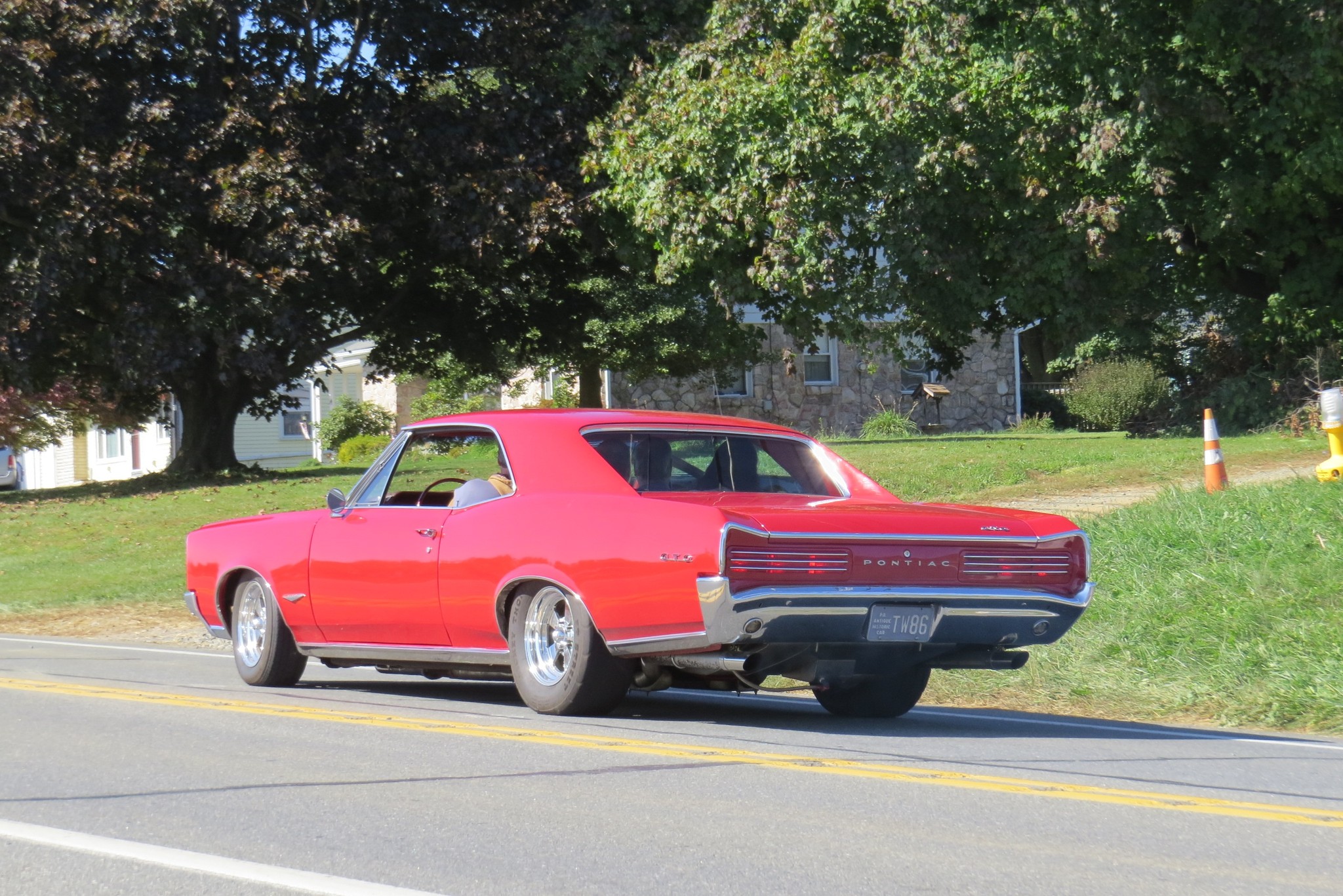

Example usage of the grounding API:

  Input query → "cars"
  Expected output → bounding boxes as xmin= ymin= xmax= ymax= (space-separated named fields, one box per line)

xmin=188 ymin=408 xmax=1091 ymax=719
xmin=0 ymin=436 xmax=27 ymax=490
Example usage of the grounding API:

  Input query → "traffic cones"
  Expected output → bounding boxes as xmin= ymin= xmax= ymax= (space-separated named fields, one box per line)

xmin=1202 ymin=408 xmax=1228 ymax=492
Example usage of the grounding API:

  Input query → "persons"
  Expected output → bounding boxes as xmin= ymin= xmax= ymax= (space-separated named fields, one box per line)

xmin=596 ymin=432 xmax=778 ymax=494
xmin=441 ymin=444 xmax=514 ymax=507
